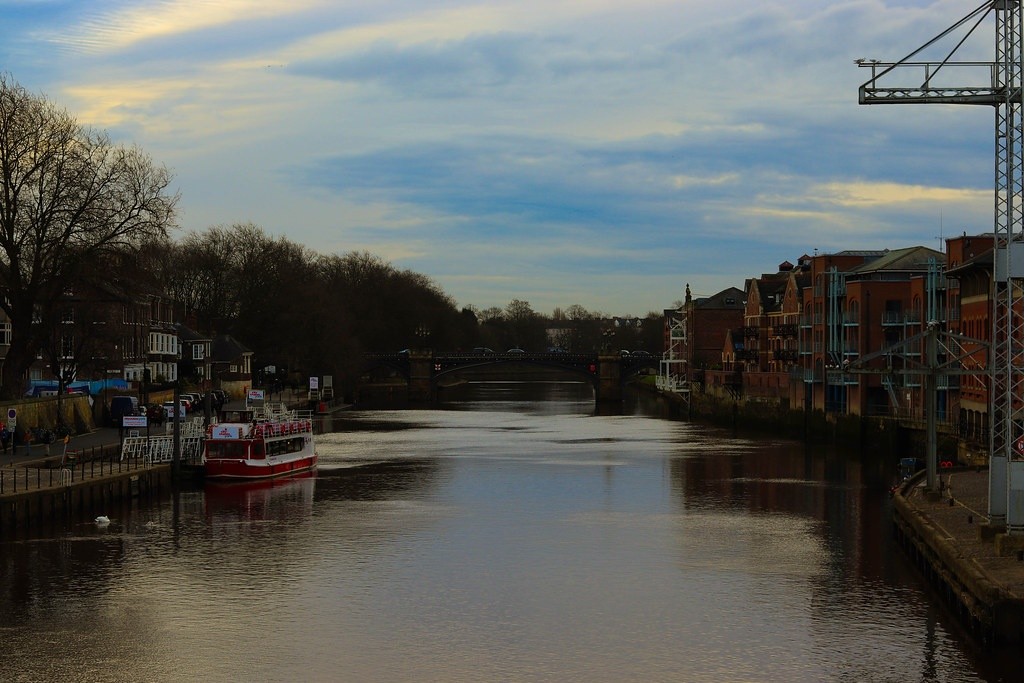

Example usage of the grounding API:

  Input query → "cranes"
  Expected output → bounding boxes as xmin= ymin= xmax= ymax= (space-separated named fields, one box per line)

xmin=857 ymin=0 xmax=1024 ymax=546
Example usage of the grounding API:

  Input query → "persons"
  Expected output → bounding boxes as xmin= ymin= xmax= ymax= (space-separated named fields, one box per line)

xmin=0 ymin=425 xmax=10 ymax=453
xmin=24 ymin=430 xmax=31 ymax=456
xmin=147 ymin=405 xmax=154 ymax=416
xmin=157 ymin=406 xmax=163 ymax=426
xmin=44 ymin=431 xmax=52 ymax=456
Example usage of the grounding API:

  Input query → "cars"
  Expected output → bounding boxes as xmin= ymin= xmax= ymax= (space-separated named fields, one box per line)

xmin=619 ymin=350 xmax=631 ymax=359
xmin=398 ymin=348 xmax=434 ymax=360
xmin=506 ymin=348 xmax=526 ymax=357
xmin=629 ymin=350 xmax=654 ymax=359
xmin=164 ymin=388 xmax=230 ymax=415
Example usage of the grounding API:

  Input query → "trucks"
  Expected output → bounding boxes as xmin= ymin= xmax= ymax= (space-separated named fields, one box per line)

xmin=547 ymin=346 xmax=571 ymax=356
xmin=110 ymin=395 xmax=142 ymax=426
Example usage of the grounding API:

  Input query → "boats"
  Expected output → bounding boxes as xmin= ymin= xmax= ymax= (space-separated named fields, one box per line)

xmin=201 ymin=401 xmax=318 ymax=482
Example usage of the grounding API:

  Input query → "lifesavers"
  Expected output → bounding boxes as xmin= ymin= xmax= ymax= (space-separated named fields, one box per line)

xmin=281 ymin=423 xmax=286 ymax=433
xmin=298 ymin=422 xmax=302 ymax=429
xmin=290 ymin=424 xmax=294 ymax=431
xmin=266 ymin=422 xmax=273 ymax=432
xmin=307 ymin=421 xmax=311 ymax=429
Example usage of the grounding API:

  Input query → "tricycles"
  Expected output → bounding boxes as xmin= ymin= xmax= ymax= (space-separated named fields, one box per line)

xmin=146 ymin=403 xmax=168 ymax=429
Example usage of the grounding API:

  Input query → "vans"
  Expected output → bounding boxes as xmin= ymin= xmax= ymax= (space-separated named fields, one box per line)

xmin=472 ymin=346 xmax=495 ymax=356
